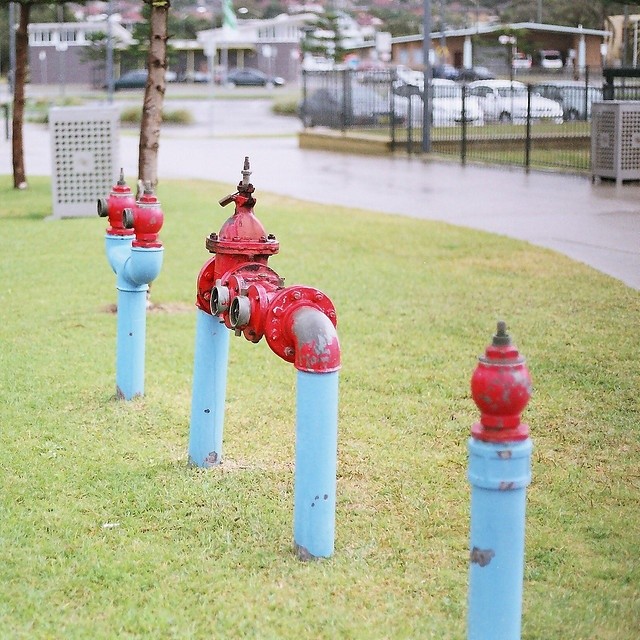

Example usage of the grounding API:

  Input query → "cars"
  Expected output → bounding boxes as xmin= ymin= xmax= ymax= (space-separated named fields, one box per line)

xmin=388 ymin=78 xmax=485 ymax=127
xmin=467 ymin=80 xmax=564 ymax=125
xmin=534 ymin=80 xmax=604 ymax=121
xmin=114 ymin=69 xmax=149 ymax=88
xmin=299 ymin=85 xmax=404 ymax=127
xmin=228 ymin=68 xmax=266 ymax=87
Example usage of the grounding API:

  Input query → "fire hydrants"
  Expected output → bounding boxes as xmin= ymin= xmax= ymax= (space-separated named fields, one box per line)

xmin=189 ymin=156 xmax=340 ymax=563
xmin=97 ymin=168 xmax=165 ymax=401
xmin=467 ymin=319 xmax=532 ymax=640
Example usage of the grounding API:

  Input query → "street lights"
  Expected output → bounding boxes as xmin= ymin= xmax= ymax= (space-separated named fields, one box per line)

xmin=600 ymin=43 xmax=608 ymax=70
xmin=499 ymin=35 xmax=515 ymax=81
xmin=629 ymin=15 xmax=640 ymax=69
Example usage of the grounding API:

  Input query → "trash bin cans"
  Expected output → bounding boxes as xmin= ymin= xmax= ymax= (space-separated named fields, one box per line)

xmin=48 ymin=107 xmax=120 ymax=218
xmin=592 ymin=68 xmax=640 ymax=186
